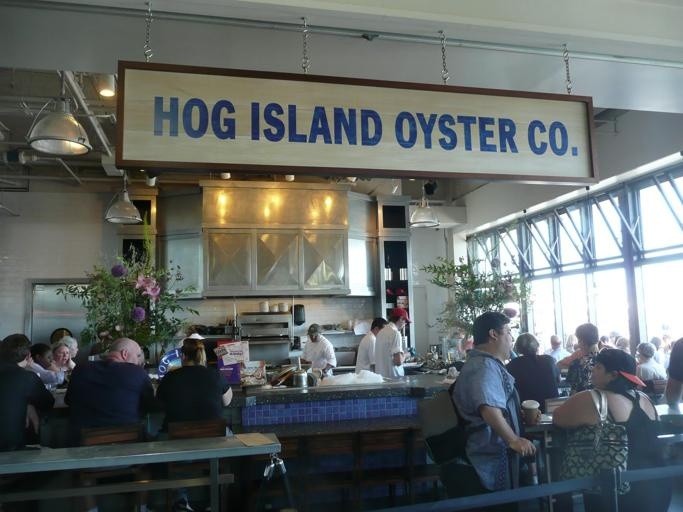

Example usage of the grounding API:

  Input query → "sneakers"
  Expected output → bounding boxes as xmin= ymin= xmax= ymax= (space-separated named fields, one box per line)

xmin=140 ymin=505 xmax=153 ymax=512
xmin=176 ymin=501 xmax=194 ymax=512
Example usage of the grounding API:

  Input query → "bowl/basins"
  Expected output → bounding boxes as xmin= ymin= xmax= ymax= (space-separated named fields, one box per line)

xmin=192 ymin=325 xmax=232 ymax=335
xmin=321 ymin=325 xmax=335 ymax=330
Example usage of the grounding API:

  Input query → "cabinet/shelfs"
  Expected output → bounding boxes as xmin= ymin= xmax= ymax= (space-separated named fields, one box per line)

xmin=319 ymin=330 xmax=365 ymax=371
xmin=379 ymin=237 xmax=416 ymax=362
xmin=181 ymin=333 xmax=235 ymax=366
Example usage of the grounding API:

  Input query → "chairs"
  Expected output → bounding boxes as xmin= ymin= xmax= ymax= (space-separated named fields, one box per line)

xmin=537 ymin=394 xmax=584 ymax=512
xmin=166 ymin=412 xmax=225 ymax=512
xmin=60 ymin=417 xmax=150 ymax=512
xmin=249 ymin=426 xmax=449 ymax=512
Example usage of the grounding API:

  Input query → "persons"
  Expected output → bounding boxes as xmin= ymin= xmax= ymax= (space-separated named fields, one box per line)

xmin=1 ymin=328 xmax=234 ymax=511
xmin=375 ymin=307 xmax=417 ymax=376
xmin=439 ymin=312 xmax=536 ymax=512
xmin=300 ymin=324 xmax=337 ymax=378
xmin=547 ymin=324 xmax=683 ymax=512
xmin=355 ymin=318 xmax=388 ymax=375
xmin=506 ymin=332 xmax=560 ymax=413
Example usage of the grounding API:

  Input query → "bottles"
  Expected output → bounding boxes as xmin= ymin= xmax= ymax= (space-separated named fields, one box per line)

xmin=386 ymin=288 xmax=408 ymax=316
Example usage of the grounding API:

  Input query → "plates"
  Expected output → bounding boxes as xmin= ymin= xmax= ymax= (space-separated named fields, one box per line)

xmin=157 ymin=348 xmax=181 ymax=380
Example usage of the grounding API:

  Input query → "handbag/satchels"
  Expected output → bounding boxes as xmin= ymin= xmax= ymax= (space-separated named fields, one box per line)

xmin=557 ymin=388 xmax=630 ymax=496
xmin=422 ymin=390 xmax=467 ymax=464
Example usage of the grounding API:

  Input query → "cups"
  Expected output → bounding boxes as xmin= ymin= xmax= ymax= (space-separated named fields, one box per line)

xmin=259 ymin=300 xmax=291 ymax=313
xmin=291 ymin=335 xmax=300 ymax=349
xmin=346 ymin=319 xmax=353 ymax=330
xmin=520 ymin=400 xmax=540 ymax=425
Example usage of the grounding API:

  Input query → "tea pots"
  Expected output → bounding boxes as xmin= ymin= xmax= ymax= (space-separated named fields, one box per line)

xmin=290 ymin=356 xmax=308 ymax=387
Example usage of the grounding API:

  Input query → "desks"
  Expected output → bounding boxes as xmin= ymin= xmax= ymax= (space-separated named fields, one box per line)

xmin=0 ymin=432 xmax=281 ymax=512
xmin=520 ymin=402 xmax=683 ymax=512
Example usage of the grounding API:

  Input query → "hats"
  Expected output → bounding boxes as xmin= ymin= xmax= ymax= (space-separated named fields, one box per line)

xmin=306 ymin=323 xmax=321 ymax=336
xmin=596 ymin=347 xmax=646 ymax=387
xmin=390 ymin=308 xmax=411 ymax=323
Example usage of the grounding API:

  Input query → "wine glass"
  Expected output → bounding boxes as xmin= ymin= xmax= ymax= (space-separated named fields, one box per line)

xmin=403 ymin=346 xmax=463 ymax=371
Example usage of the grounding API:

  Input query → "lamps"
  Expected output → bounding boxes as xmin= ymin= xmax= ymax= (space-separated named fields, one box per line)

xmin=98 ymin=73 xmax=115 ymax=97
xmin=106 ymin=168 xmax=143 ymax=226
xmin=24 ymin=71 xmax=92 ymax=157
xmin=408 ymin=178 xmax=439 ymax=228
